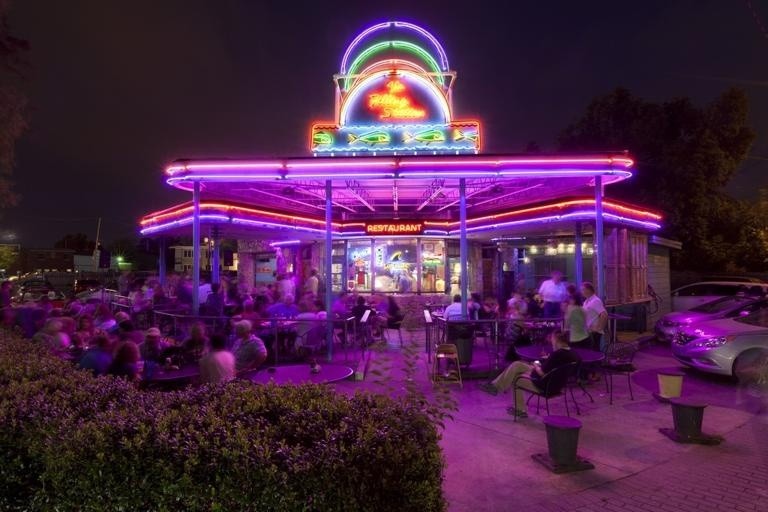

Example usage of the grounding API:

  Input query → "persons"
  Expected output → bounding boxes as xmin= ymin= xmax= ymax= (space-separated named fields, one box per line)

xmin=442 ymin=270 xmax=608 ymax=419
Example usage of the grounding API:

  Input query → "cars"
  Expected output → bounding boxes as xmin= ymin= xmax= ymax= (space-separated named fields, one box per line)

xmin=654 ymin=291 xmax=768 ymax=344
xmin=0 ymin=270 xmax=148 ymax=304
xmin=671 ymin=307 xmax=768 ymax=389
xmin=670 ymin=281 xmax=768 ymax=312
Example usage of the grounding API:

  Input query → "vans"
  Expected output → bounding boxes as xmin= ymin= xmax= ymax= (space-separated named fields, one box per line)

xmin=671 ymin=274 xmax=764 ymax=291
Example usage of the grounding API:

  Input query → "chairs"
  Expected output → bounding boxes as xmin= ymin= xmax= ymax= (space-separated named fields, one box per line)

xmin=432 ymin=343 xmax=463 ymax=389
xmin=604 ymin=341 xmax=635 ymax=405
xmin=567 ymin=361 xmax=595 ymax=415
xmin=447 ymin=315 xmax=466 ymax=322
xmin=7 ymin=281 xmax=405 ymax=389
xmin=514 ymin=365 xmax=571 ymax=417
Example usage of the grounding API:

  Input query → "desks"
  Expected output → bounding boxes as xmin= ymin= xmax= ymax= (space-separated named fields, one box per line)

xmin=517 ymin=345 xmax=606 ymax=416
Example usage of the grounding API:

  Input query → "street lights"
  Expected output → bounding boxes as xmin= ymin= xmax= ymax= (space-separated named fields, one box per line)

xmin=204 ymin=237 xmax=215 ymax=277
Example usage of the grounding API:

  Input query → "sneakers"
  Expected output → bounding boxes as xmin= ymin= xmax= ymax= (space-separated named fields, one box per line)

xmin=510 ymin=409 xmax=528 ymax=418
xmin=474 ymin=383 xmax=498 ymax=396
